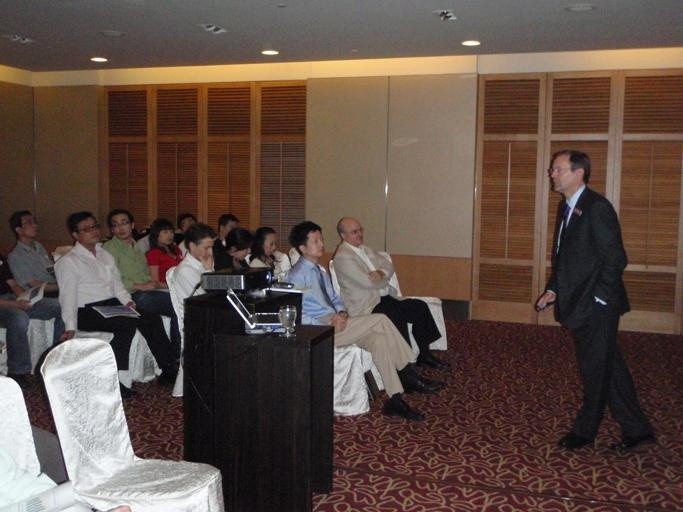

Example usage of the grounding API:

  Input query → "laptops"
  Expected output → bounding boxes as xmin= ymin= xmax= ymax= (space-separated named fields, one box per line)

xmin=223 ymin=290 xmax=296 ymax=329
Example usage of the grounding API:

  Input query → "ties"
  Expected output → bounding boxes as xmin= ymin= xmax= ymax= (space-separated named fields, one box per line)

xmin=560 ymin=205 xmax=570 ymax=243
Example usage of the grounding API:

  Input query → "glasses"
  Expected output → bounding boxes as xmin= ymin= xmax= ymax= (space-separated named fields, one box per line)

xmin=78 ymin=223 xmax=100 ymax=232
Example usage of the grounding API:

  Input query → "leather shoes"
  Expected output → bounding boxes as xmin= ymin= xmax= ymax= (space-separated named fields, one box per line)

xmin=561 ymin=428 xmax=594 ymax=447
xmin=613 ymin=434 xmax=657 ymax=448
xmin=404 ymin=376 xmax=442 ymax=393
xmin=416 ymin=355 xmax=449 ymax=369
xmin=382 ymin=393 xmax=424 ymax=421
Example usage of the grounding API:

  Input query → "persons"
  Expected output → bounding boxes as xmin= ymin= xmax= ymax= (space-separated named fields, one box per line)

xmin=284 ymin=209 xmax=451 ymax=420
xmin=534 ymin=149 xmax=657 ymax=450
xmin=0 ymin=209 xmax=279 ymax=394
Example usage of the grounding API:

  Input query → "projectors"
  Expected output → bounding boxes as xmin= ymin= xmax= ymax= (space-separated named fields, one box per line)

xmin=201 ymin=266 xmax=276 ymax=290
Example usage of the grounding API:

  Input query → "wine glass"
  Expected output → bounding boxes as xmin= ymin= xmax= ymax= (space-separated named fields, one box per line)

xmin=276 ymin=304 xmax=298 ymax=339
